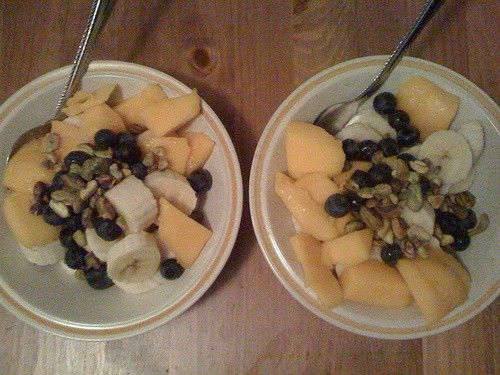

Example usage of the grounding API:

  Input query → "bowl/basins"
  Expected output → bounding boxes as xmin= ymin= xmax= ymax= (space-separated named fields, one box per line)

xmin=249 ymin=55 xmax=499 ymax=341
xmin=0 ymin=61 xmax=245 ymax=342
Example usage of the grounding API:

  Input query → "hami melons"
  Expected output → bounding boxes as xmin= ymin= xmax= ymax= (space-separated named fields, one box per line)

xmin=6 ymin=82 xmax=217 ymax=285
xmin=274 ymin=75 xmax=487 ymax=323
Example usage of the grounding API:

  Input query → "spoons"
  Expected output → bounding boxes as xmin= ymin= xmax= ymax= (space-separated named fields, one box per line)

xmin=7 ymin=0 xmax=117 ymax=167
xmin=306 ymin=0 xmax=446 ymax=136
